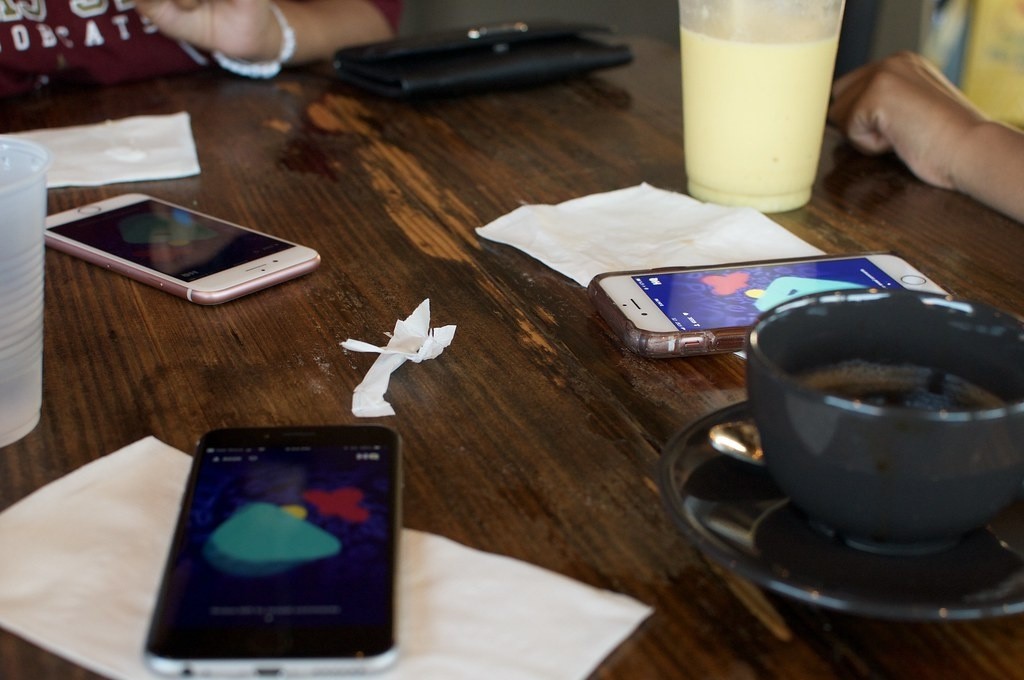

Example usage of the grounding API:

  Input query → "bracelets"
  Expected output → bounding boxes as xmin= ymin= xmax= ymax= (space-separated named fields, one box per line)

xmin=210 ymin=0 xmax=296 ymax=79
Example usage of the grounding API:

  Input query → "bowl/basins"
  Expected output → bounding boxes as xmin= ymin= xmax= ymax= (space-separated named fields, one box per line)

xmin=747 ymin=287 xmax=1024 ymax=552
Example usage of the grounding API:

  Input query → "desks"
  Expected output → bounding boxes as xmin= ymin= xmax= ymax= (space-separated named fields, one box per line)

xmin=0 ymin=0 xmax=1024 ymax=680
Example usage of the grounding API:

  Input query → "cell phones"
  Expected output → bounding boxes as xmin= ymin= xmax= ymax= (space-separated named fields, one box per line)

xmin=142 ymin=421 xmax=403 ymax=676
xmin=588 ymin=252 xmax=951 ymax=360
xmin=46 ymin=193 xmax=321 ymax=307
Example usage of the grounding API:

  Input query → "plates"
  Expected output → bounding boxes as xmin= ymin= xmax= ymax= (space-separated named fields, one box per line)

xmin=656 ymin=397 xmax=1024 ymax=620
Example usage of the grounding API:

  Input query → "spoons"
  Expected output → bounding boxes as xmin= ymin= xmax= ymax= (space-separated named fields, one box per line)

xmin=705 ymin=419 xmax=769 ymax=468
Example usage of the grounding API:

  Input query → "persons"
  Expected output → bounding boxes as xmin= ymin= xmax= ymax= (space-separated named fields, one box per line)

xmin=0 ymin=0 xmax=395 ymax=97
xmin=824 ymin=48 xmax=1024 ymax=225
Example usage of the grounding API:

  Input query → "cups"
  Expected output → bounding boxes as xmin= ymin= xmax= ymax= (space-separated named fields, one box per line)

xmin=680 ymin=1 xmax=845 ymax=215
xmin=0 ymin=133 xmax=52 ymax=446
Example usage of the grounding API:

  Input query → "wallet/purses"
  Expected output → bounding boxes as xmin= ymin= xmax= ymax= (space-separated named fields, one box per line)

xmin=332 ymin=18 xmax=634 ymax=100
